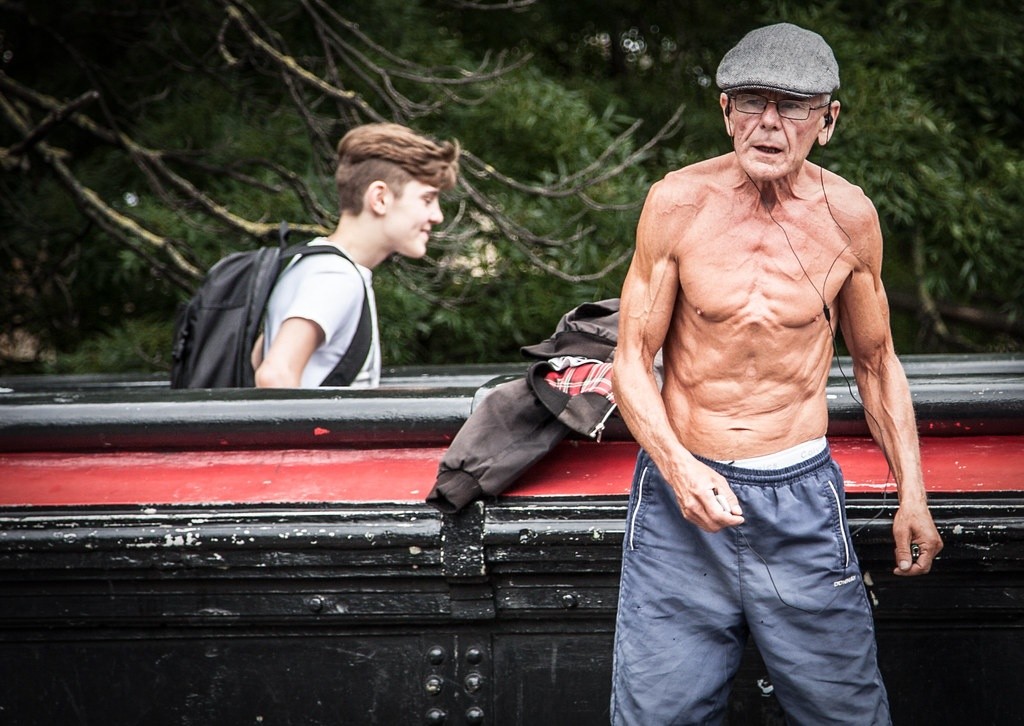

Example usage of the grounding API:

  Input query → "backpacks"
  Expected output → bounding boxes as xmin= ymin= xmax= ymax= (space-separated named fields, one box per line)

xmin=170 ymin=221 xmax=373 ymax=390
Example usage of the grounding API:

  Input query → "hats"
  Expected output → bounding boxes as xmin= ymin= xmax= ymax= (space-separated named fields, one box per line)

xmin=716 ymin=22 xmax=840 ymax=98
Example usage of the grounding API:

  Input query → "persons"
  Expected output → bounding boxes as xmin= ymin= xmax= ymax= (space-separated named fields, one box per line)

xmin=250 ymin=122 xmax=460 ymax=387
xmin=609 ymin=23 xmax=943 ymax=726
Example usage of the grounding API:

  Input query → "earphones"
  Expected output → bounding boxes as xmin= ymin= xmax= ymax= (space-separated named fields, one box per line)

xmin=724 ymin=106 xmax=731 ymax=117
xmin=823 ymin=114 xmax=833 ymax=128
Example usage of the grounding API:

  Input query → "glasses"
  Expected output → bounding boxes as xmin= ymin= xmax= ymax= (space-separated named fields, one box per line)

xmin=728 ymin=92 xmax=830 ymax=120
